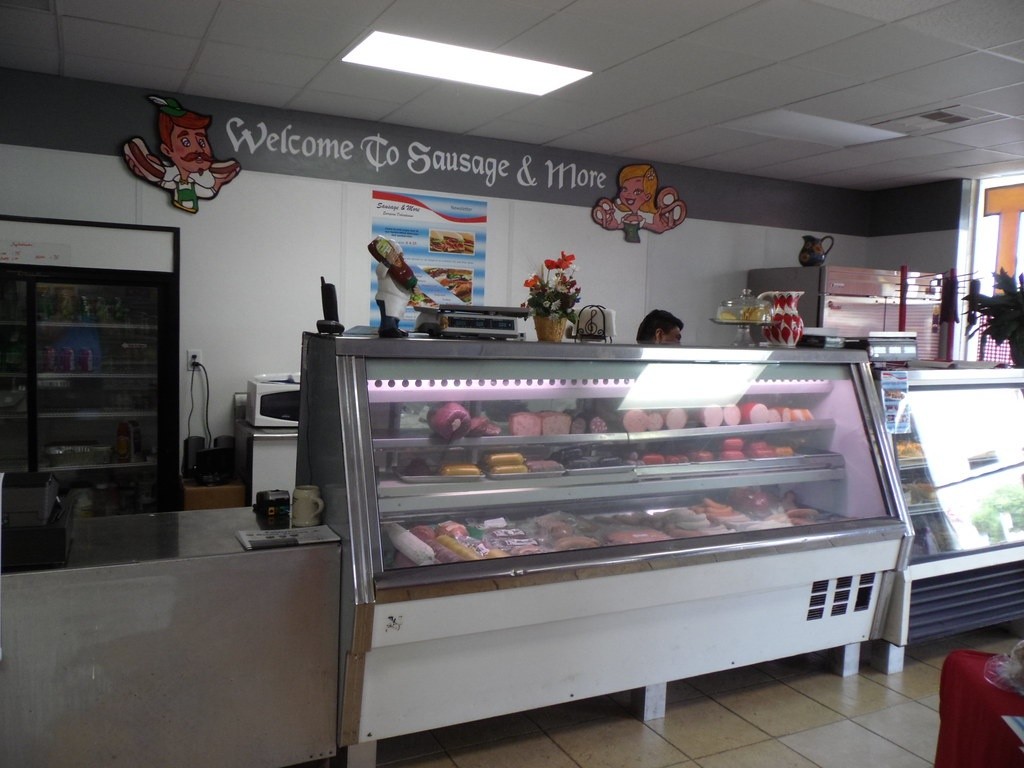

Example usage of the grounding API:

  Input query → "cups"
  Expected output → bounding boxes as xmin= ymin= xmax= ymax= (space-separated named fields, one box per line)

xmin=290 ymin=484 xmax=324 ymax=528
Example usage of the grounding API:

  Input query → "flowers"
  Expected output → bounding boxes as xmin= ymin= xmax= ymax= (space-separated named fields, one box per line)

xmin=522 ymin=252 xmax=582 ymax=326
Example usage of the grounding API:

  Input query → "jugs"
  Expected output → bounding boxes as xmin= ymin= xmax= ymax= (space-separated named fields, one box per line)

xmin=799 ymin=235 xmax=834 ymax=266
xmin=755 ymin=290 xmax=806 ymax=347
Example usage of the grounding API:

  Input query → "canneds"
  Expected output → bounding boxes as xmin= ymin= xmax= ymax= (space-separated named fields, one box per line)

xmin=41 ymin=346 xmax=96 ymax=373
xmin=35 ymin=291 xmax=129 ymax=322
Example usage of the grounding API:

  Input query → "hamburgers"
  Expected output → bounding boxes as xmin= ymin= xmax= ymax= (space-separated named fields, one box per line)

xmin=429 ymin=230 xmax=474 ymax=255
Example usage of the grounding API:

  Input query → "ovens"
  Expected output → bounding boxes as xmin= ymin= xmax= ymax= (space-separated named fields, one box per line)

xmin=245 ymin=379 xmax=301 ymax=429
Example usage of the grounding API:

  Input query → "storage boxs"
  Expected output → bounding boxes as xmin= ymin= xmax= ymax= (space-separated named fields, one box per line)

xmin=181 ymin=476 xmax=245 ymax=510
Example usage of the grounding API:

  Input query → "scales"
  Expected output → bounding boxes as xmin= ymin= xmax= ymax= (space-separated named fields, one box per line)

xmin=797 ymin=327 xmax=918 ymax=368
xmin=414 ymin=304 xmax=530 ymax=340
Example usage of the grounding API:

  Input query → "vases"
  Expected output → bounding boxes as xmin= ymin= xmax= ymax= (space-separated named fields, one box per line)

xmin=532 ymin=316 xmax=567 ymax=342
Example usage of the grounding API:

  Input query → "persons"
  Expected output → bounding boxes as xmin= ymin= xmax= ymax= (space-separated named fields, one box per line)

xmin=636 ymin=309 xmax=683 ymax=345
xmin=376 ymin=239 xmax=420 ymax=338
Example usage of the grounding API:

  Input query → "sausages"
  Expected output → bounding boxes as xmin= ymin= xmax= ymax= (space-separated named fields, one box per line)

xmin=385 ymin=402 xmax=814 ymax=566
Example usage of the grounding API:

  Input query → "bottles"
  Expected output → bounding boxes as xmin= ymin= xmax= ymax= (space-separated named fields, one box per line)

xmin=5 ymin=333 xmax=27 ymax=371
xmin=367 ymin=235 xmax=418 ymax=290
xmin=717 ymin=288 xmax=754 ymax=320
xmin=116 ymin=418 xmax=142 ymax=463
xmin=93 ymin=481 xmax=121 ymax=515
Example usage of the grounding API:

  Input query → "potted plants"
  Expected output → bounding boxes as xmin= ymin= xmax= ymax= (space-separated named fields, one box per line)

xmin=961 ymin=268 xmax=1024 ymax=366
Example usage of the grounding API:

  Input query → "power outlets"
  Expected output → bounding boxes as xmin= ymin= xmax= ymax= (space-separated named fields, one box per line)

xmin=187 ymin=349 xmax=203 ymax=371
